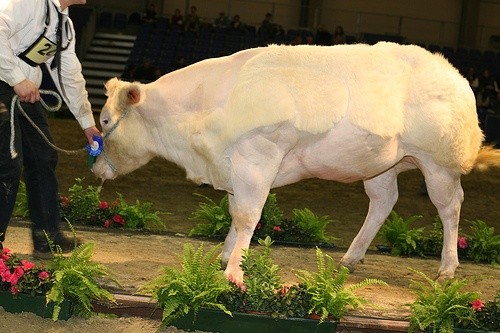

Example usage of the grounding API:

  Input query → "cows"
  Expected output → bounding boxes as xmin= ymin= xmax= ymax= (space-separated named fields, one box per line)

xmin=89 ymin=40 xmax=500 ymax=292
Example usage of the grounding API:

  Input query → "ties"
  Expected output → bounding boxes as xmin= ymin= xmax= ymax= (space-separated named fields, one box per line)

xmin=51 ymin=2 xmax=71 ymax=104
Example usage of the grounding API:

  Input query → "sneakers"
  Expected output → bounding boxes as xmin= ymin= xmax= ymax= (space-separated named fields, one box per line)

xmin=44 ymin=234 xmax=84 ymax=253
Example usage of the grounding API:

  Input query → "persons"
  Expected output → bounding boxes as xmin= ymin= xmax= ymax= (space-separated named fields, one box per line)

xmin=135 ymin=2 xmax=347 ymax=80
xmin=460 ymin=67 xmax=500 ymax=131
xmin=0 ymin=1 xmax=103 ymax=259
